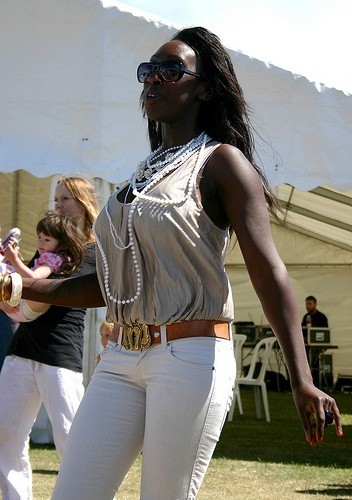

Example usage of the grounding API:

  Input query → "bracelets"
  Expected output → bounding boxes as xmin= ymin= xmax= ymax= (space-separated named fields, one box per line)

xmin=1 ymin=273 xmax=22 ymax=308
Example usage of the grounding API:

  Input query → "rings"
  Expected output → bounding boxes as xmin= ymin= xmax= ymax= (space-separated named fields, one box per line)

xmin=325 ymin=410 xmax=333 ymax=424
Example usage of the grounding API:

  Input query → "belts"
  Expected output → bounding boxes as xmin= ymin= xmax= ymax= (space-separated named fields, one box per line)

xmin=109 ymin=318 xmax=230 ymax=351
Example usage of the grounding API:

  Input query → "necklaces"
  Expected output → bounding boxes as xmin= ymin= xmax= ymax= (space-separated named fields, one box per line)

xmin=92 ymin=131 xmax=210 ymax=304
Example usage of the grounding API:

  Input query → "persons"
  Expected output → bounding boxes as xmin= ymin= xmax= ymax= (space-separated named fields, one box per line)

xmin=0 ymin=27 xmax=344 ymax=500
xmin=0 ymin=178 xmax=99 ymax=500
xmin=0 ymin=216 xmax=83 ymax=323
xmin=301 ymin=296 xmax=330 ymax=366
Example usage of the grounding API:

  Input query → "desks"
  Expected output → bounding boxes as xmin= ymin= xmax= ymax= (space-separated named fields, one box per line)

xmin=242 ymin=340 xmax=338 ymax=392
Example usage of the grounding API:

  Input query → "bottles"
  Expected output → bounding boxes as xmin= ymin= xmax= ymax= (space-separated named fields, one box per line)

xmin=0 ymin=227 xmax=21 ymax=255
xmin=305 ymin=315 xmax=311 ymax=327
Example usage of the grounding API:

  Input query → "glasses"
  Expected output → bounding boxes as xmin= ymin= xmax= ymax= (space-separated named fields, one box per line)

xmin=136 ymin=59 xmax=200 ymax=84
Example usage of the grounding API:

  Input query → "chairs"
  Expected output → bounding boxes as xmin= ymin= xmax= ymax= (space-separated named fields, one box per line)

xmin=227 ymin=334 xmax=276 ymax=422
